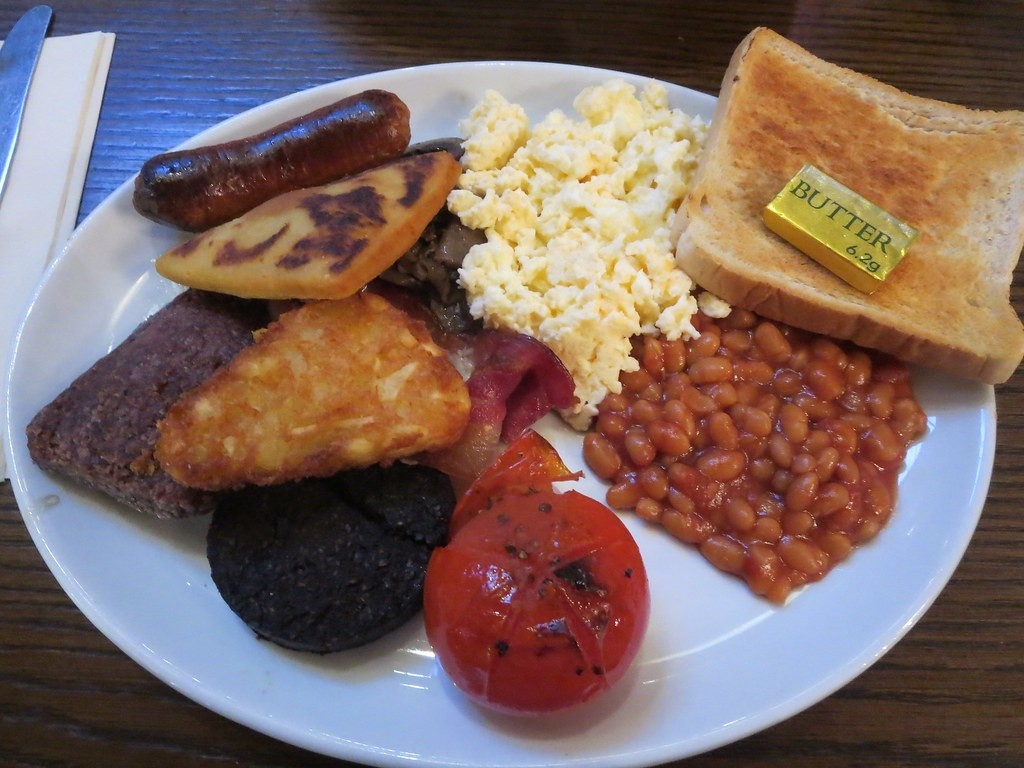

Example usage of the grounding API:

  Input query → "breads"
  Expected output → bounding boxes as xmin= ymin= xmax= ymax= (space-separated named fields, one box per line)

xmin=207 ymin=463 xmax=455 ymax=651
xmin=674 ymin=24 xmax=1024 ymax=385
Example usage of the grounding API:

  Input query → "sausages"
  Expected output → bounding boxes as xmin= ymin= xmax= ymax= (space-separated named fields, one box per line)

xmin=133 ymin=87 xmax=410 ymax=231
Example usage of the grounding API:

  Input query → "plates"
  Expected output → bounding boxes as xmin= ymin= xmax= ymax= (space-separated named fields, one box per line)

xmin=1 ymin=59 xmax=997 ymax=768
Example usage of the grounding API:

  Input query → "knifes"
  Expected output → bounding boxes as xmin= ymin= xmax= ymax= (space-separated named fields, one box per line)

xmin=1 ymin=7 xmax=53 ymax=199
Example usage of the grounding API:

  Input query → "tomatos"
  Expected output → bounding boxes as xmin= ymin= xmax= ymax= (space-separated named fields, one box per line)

xmin=420 ymin=428 xmax=650 ymax=717
